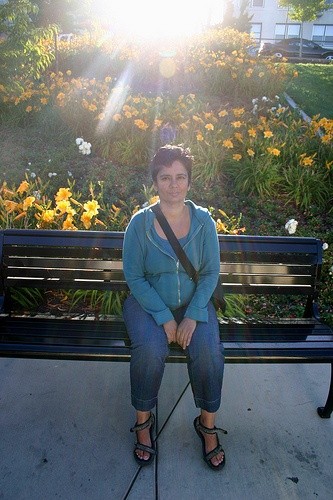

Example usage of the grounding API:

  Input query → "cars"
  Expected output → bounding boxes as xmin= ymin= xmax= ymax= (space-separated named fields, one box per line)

xmin=260 ymin=38 xmax=333 ymax=64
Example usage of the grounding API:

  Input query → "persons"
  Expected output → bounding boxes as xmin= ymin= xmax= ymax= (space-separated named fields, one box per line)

xmin=121 ymin=144 xmax=229 ymax=471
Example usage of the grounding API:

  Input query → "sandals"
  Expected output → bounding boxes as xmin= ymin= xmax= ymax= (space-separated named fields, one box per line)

xmin=193 ymin=414 xmax=228 ymax=471
xmin=129 ymin=410 xmax=156 ymax=465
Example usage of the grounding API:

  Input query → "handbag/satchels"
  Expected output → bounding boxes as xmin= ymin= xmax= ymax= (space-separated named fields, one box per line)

xmin=209 ymin=275 xmax=226 ymax=314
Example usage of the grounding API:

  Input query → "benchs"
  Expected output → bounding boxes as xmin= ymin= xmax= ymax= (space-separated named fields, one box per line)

xmin=0 ymin=230 xmax=333 ymax=420
